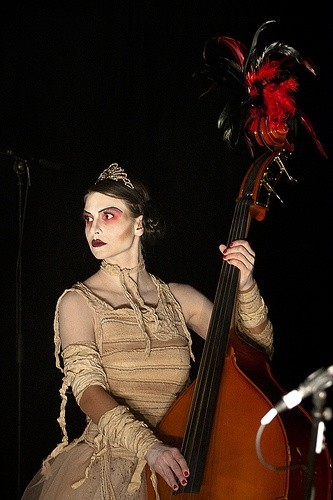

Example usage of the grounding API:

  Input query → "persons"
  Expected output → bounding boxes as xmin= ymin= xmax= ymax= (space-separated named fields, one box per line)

xmin=18 ymin=161 xmax=276 ymax=499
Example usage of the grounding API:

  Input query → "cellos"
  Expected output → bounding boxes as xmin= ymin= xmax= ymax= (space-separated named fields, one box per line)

xmin=143 ymin=131 xmax=332 ymax=499
